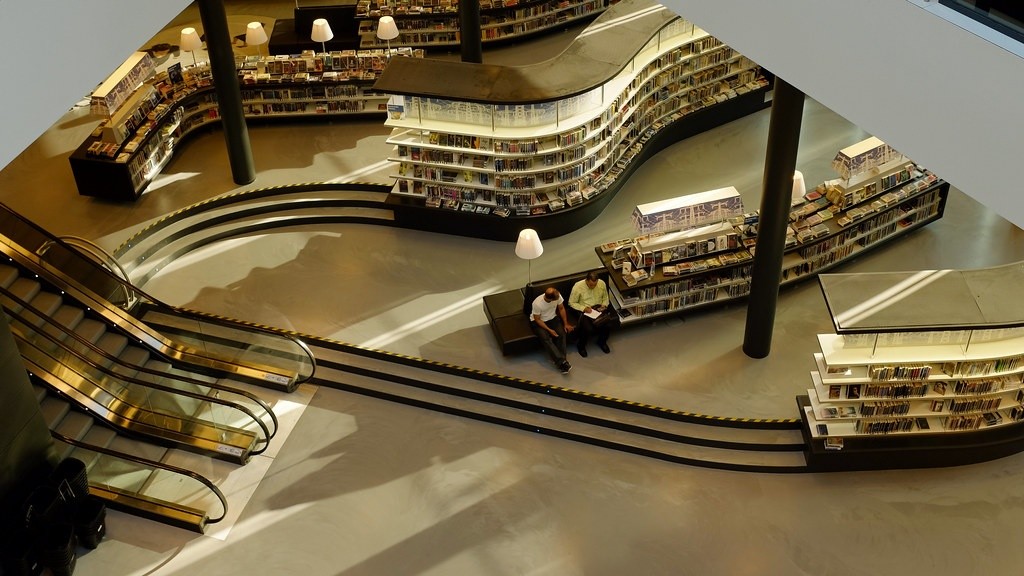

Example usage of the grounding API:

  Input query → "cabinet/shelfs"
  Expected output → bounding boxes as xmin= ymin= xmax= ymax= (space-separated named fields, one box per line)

xmin=795 ymin=259 xmax=1024 ymax=471
xmin=67 ymin=47 xmax=424 ymax=202
xmin=353 ymin=0 xmax=620 ymax=55
xmin=371 ymin=0 xmax=776 ymax=242
xmin=593 ymin=135 xmax=949 ymax=327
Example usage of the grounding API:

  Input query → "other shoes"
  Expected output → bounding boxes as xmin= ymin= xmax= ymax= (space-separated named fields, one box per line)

xmin=597 ymin=341 xmax=610 ymax=354
xmin=560 ymin=360 xmax=572 ymax=375
xmin=577 ymin=344 xmax=587 ymax=357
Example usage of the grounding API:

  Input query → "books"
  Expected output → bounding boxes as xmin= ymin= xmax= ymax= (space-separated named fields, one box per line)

xmin=600 ymin=163 xmax=942 ymax=319
xmin=88 ymin=48 xmax=424 ymax=190
xmin=386 ymin=32 xmax=770 ymax=217
xmin=356 ymin=0 xmax=620 ymax=47
xmin=810 ymin=357 xmax=1024 ymax=450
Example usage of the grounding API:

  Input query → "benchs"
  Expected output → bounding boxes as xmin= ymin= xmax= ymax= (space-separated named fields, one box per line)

xmin=268 ymin=4 xmax=360 ymax=56
xmin=482 ymin=267 xmax=620 ymax=355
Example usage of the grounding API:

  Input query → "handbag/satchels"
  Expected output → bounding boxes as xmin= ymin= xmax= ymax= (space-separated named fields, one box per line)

xmin=586 ymin=304 xmax=610 ymax=329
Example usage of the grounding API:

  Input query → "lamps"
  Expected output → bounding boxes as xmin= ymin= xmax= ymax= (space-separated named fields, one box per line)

xmin=246 ymin=22 xmax=268 ymax=61
xmin=376 ymin=16 xmax=399 ymax=58
xmin=310 ymin=18 xmax=334 ymax=58
xmin=180 ymin=27 xmax=202 ymax=75
xmin=791 ymin=170 xmax=806 ymax=199
xmin=513 ymin=229 xmax=544 ymax=287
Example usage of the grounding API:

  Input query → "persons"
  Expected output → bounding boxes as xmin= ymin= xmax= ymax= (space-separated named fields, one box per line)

xmin=568 ymin=272 xmax=610 ymax=357
xmin=530 ymin=288 xmax=574 ymax=374
